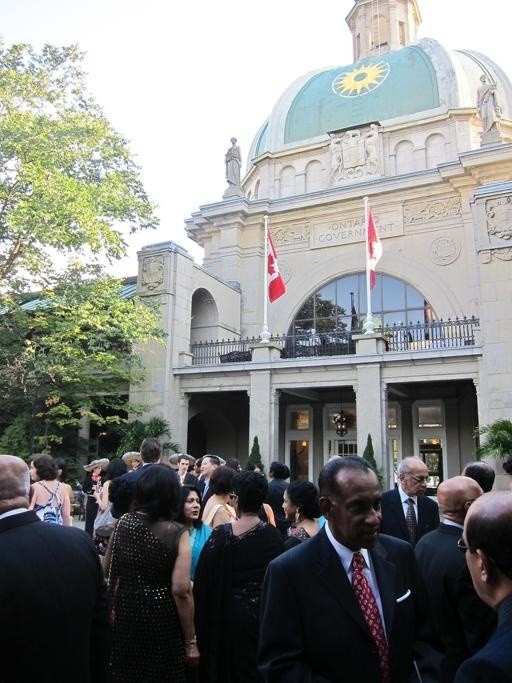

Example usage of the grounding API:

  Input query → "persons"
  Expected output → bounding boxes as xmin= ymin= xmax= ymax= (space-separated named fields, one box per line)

xmin=473 ymin=72 xmax=502 ymax=148
xmin=219 ymin=136 xmax=245 ymax=199
xmin=1 ymin=437 xmax=512 ymax=682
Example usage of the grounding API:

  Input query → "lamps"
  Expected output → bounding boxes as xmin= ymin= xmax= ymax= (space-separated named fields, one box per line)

xmin=334 ymin=399 xmax=350 ymax=437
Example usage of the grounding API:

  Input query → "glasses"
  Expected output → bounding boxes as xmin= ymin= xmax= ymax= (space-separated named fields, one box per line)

xmin=456 ymin=538 xmax=474 ymax=552
xmin=408 ymin=474 xmax=430 ymax=482
xmin=457 ymin=537 xmax=471 ymax=551
xmin=229 ymin=491 xmax=237 ymax=500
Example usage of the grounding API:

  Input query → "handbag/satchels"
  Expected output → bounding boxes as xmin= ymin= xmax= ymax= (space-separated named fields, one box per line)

xmin=93 ymin=502 xmax=117 ymax=536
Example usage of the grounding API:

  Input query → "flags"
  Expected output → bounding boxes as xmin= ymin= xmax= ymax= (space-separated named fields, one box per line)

xmin=368 ymin=206 xmax=387 ymax=291
xmin=351 ymin=304 xmax=359 ymax=334
xmin=267 ymin=229 xmax=290 ymax=305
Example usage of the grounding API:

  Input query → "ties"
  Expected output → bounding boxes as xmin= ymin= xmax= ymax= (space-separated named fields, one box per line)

xmin=404 ymin=499 xmax=417 ymax=544
xmin=350 ymin=552 xmax=392 ymax=682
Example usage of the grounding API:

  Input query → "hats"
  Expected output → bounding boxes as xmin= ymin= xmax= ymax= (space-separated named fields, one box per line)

xmin=169 ymin=454 xmax=196 ymax=466
xmin=82 ymin=457 xmax=110 ymax=472
xmin=122 ymin=451 xmax=143 ymax=465
